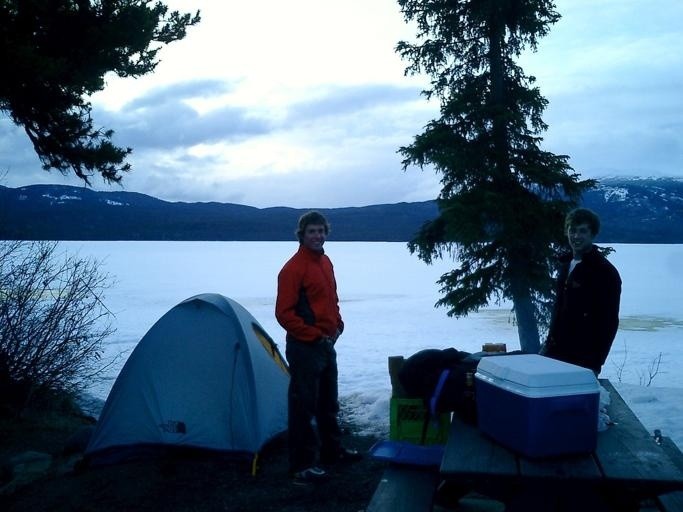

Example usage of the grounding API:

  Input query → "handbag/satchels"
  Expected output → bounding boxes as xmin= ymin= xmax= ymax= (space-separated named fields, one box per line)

xmin=458 ymin=351 xmax=529 ymax=424
xmin=398 ymin=347 xmax=470 ymax=421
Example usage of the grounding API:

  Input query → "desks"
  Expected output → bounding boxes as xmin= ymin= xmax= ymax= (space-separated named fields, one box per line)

xmin=438 ymin=378 xmax=682 ymax=512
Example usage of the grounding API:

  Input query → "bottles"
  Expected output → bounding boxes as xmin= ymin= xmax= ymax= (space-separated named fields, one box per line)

xmin=654 ymin=429 xmax=662 ymax=446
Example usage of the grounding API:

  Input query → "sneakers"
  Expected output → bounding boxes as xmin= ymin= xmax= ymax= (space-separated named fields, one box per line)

xmin=323 ymin=447 xmax=359 ymax=462
xmin=293 ymin=467 xmax=328 ymax=486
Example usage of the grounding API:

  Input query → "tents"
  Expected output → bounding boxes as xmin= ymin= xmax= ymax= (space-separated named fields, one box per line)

xmin=73 ymin=293 xmax=290 ymax=476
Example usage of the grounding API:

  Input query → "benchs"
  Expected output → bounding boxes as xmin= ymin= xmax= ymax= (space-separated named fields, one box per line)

xmin=645 ymin=434 xmax=682 ymax=512
xmin=361 ymin=375 xmax=452 ymax=512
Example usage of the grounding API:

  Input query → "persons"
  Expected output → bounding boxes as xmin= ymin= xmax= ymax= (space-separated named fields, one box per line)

xmin=274 ymin=213 xmax=359 ymax=483
xmin=541 ymin=207 xmax=621 ymax=377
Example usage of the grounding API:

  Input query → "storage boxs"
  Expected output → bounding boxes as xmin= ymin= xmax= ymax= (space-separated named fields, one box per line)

xmin=387 ymin=388 xmax=449 ymax=445
xmin=472 ymin=353 xmax=604 ymax=463
